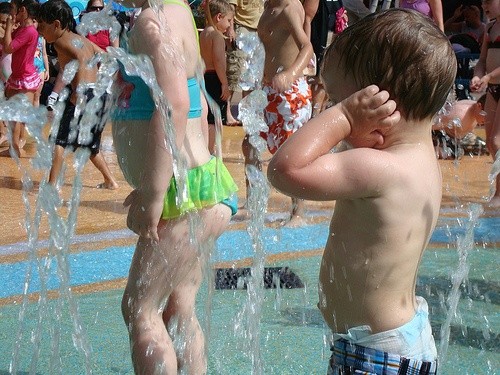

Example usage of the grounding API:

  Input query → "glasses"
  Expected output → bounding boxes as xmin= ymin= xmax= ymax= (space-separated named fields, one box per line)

xmin=90 ymin=6 xmax=104 ymax=11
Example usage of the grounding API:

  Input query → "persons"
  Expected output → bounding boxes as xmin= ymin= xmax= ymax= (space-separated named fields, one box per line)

xmin=110 ymin=0 xmax=238 ymax=375
xmin=0 ymin=0 xmax=141 ymax=191
xmin=199 ymin=0 xmax=500 ymax=228
xmin=267 ymin=8 xmax=457 ymax=375
